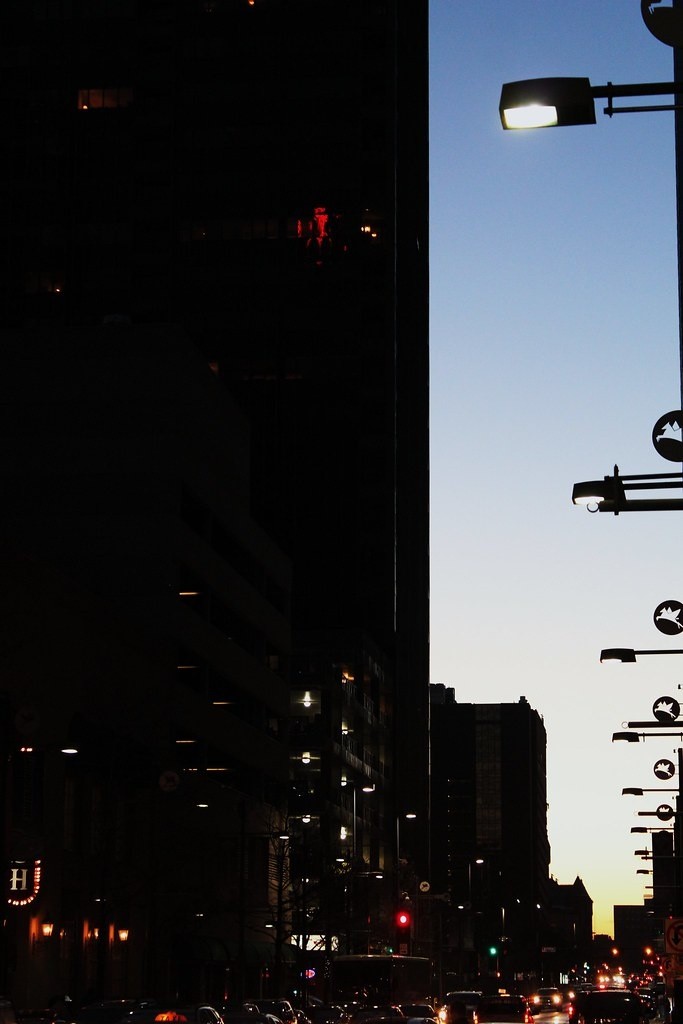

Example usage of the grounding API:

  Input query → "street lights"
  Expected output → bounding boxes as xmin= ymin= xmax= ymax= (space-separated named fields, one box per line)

xmin=352 ymin=782 xmax=376 ymax=856
xmin=468 ymin=856 xmax=485 ymax=903
xmin=397 ymin=808 xmax=417 ymax=892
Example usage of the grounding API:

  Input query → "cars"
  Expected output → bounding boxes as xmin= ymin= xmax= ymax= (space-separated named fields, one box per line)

xmin=2 ymin=945 xmax=683 ymax=1024
